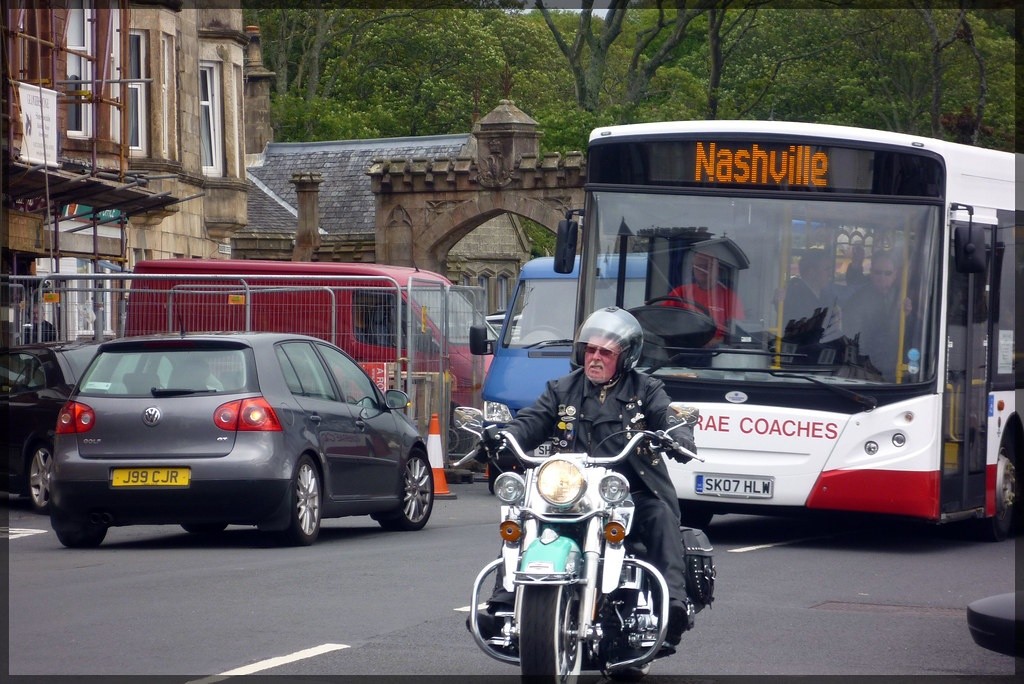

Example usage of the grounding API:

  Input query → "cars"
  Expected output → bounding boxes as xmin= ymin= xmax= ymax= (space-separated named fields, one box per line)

xmin=48 ymin=332 xmax=436 ymax=548
xmin=0 ymin=338 xmax=126 ymax=514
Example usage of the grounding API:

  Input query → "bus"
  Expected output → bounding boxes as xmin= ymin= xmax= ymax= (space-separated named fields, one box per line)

xmin=554 ymin=118 xmax=1022 ymax=545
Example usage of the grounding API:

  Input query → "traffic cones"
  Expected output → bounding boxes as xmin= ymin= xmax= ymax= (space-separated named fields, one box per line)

xmin=474 ymin=462 xmax=490 ymax=482
xmin=422 ymin=413 xmax=457 ymax=499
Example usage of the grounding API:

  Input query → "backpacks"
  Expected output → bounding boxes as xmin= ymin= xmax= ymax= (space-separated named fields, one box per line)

xmin=679 ymin=525 xmax=715 ymax=614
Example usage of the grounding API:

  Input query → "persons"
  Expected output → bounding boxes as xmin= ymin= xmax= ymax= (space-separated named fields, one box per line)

xmin=656 ymin=250 xmax=742 ymax=346
xmin=849 ymin=252 xmax=911 ymax=365
xmin=775 ymin=247 xmax=831 ymax=362
xmin=464 ymin=306 xmax=695 ymax=645
xmin=845 ymin=245 xmax=910 ymax=286
xmin=23 ymin=303 xmax=56 ymax=342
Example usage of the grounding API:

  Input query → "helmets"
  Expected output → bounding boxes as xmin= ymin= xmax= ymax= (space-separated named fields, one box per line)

xmin=569 ymin=306 xmax=644 ymax=389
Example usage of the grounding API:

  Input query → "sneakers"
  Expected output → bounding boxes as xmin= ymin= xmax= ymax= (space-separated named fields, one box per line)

xmin=657 ymin=598 xmax=689 ymax=647
xmin=465 ymin=602 xmax=519 ymax=659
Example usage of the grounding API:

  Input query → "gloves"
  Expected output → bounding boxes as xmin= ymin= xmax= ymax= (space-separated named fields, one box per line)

xmin=471 ymin=425 xmax=511 ymax=464
xmin=666 ymin=424 xmax=698 ymax=464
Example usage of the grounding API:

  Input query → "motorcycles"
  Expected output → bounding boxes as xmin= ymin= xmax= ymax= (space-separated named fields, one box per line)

xmin=450 ymin=403 xmax=719 ymax=683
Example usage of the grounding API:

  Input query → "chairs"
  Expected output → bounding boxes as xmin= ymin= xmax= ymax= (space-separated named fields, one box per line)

xmin=123 ymin=369 xmax=251 ymax=394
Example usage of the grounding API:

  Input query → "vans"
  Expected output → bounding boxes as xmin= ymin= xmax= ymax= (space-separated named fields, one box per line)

xmin=124 ymin=256 xmax=511 ymax=467
xmin=470 ymin=251 xmax=650 ymax=496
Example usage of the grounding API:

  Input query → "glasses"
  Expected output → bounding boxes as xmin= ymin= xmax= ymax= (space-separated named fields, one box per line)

xmin=870 ymin=268 xmax=895 ymax=276
xmin=583 ymin=346 xmax=615 ymax=356
